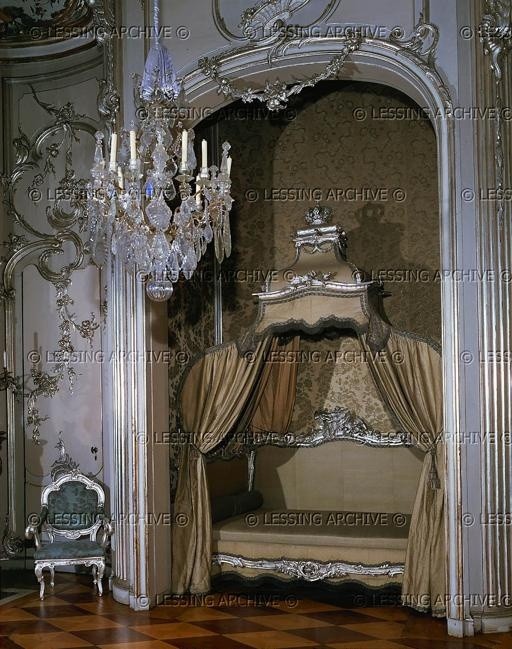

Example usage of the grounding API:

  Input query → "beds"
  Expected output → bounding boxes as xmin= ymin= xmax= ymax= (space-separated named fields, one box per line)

xmin=205 ymin=407 xmax=427 ymax=592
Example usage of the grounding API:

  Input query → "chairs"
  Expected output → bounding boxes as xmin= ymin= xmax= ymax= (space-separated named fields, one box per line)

xmin=25 ymin=468 xmax=112 ymax=602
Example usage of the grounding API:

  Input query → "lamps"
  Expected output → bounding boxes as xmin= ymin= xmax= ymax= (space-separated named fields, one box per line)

xmin=87 ymin=0 xmax=236 ymax=305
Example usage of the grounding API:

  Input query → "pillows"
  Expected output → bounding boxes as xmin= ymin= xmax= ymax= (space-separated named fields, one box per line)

xmin=210 ymin=490 xmax=264 ymax=523
